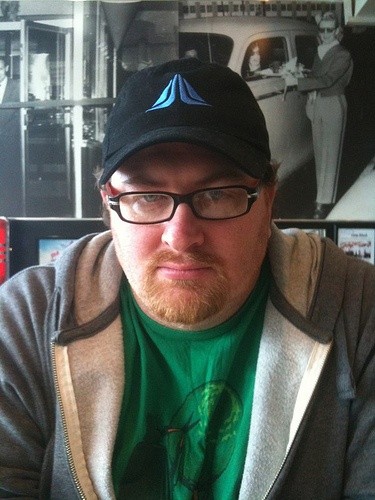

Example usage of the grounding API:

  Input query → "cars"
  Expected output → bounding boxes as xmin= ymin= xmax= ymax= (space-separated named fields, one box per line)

xmin=177 ymin=15 xmax=323 ymax=192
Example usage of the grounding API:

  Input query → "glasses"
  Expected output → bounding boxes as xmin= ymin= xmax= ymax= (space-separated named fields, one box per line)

xmin=107 ymin=182 xmax=263 ymax=224
xmin=319 ymin=27 xmax=337 ymax=33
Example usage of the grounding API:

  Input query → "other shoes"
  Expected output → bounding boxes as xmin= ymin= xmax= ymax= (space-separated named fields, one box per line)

xmin=312 ymin=202 xmax=334 ymax=220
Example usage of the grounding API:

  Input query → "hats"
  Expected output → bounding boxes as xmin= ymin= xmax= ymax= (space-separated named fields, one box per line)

xmin=99 ymin=57 xmax=272 ymax=186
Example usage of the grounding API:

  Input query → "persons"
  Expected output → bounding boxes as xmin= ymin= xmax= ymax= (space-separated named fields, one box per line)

xmin=282 ymin=11 xmax=353 ymax=220
xmin=0 ymin=59 xmax=19 ymax=135
xmin=242 ymin=43 xmax=281 ymax=81
xmin=0 ymin=58 xmax=375 ymax=500
